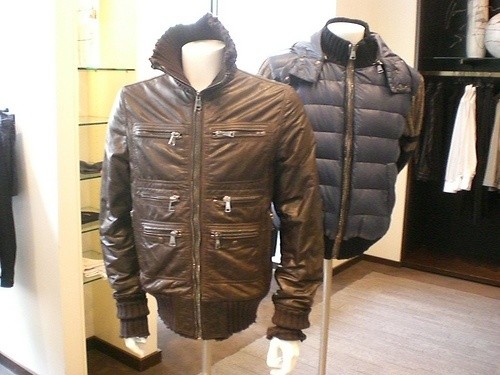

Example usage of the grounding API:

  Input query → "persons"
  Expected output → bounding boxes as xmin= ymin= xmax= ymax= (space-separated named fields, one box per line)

xmin=97 ymin=12 xmax=325 ymax=375
xmin=256 ymin=18 xmax=425 ymax=262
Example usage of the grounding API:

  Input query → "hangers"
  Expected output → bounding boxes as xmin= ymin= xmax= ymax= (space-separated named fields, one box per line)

xmin=428 ymin=70 xmax=495 ymax=87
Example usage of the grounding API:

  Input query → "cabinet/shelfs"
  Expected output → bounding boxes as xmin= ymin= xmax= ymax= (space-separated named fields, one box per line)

xmin=77 ymin=66 xmax=135 ymax=284
